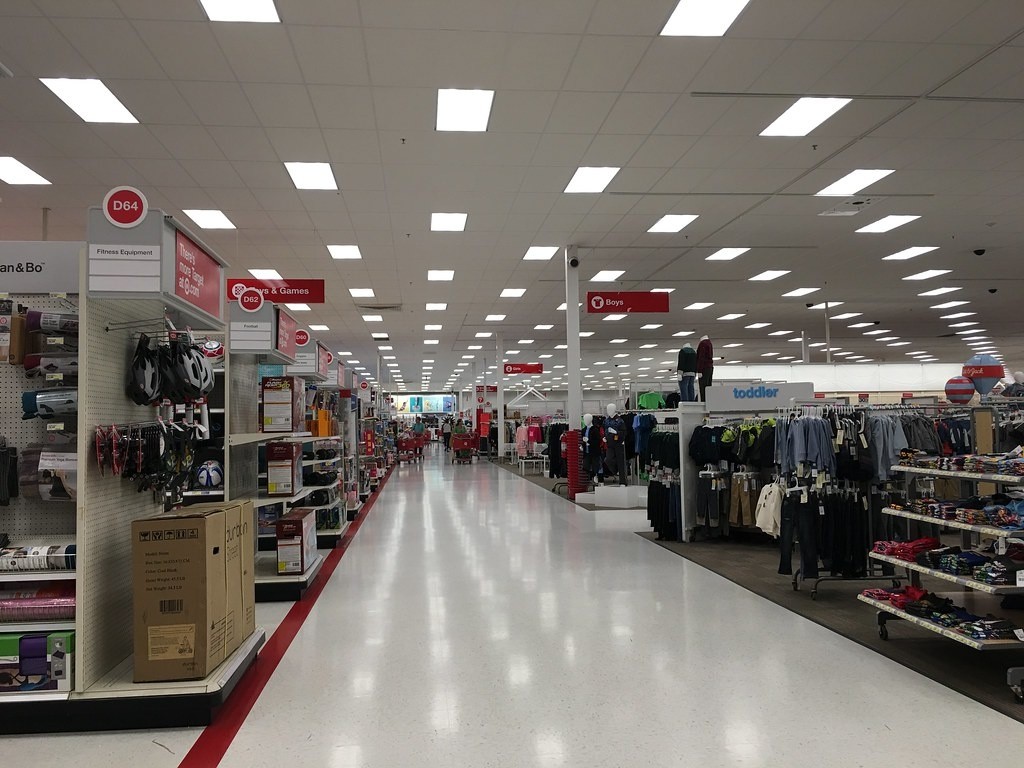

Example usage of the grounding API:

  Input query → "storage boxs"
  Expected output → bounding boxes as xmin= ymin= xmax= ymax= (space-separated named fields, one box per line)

xmin=131 ymin=509 xmax=225 ymax=684
xmin=184 ymin=500 xmax=256 ymax=641
xmin=276 ymin=507 xmax=318 ymax=576
xmin=172 ymin=505 xmax=241 ymax=660
xmin=267 ymin=442 xmax=303 ymax=497
xmin=261 ymin=376 xmax=306 ymax=432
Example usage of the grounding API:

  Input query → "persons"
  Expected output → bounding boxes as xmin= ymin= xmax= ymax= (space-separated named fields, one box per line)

xmin=425 ymin=421 xmax=429 ymax=429
xmin=603 ymin=402 xmax=629 ymax=488
xmin=412 ymin=416 xmax=424 ymax=463
xmin=442 ymin=418 xmax=452 ymax=453
xmin=1001 ymin=372 xmax=1024 ymax=396
xmin=579 ymin=412 xmax=604 ymax=487
xmin=696 ymin=334 xmax=714 ymax=402
xmin=677 ymin=342 xmax=696 ymax=402
xmin=453 ymin=418 xmax=468 ymax=464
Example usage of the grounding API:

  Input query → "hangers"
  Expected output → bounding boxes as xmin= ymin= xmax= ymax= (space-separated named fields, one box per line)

xmin=519 ymin=419 xmax=547 ymax=430
xmin=743 ymin=416 xmax=768 ymax=429
xmin=929 ymin=410 xmax=1024 ymax=429
xmin=618 ymin=408 xmax=677 ymax=416
xmin=550 ymin=418 xmax=570 ymax=425
xmin=733 ymin=465 xmax=757 ymax=479
xmin=699 ymin=464 xmax=728 ymax=479
xmin=702 ymin=416 xmax=739 ymax=429
xmin=768 ymin=473 xmax=934 ymax=498
xmin=653 ymin=424 xmax=680 ymax=433
xmin=648 ymin=469 xmax=681 ymax=486
xmin=776 ymin=404 xmax=921 ymax=425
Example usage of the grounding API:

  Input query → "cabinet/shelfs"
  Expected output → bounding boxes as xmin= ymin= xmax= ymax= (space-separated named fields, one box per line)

xmin=258 ymin=337 xmax=354 ymax=550
xmin=339 ymin=368 xmax=364 ymax=519
xmin=0 ymin=190 xmax=266 ymax=738
xmin=856 ymin=463 xmax=1024 ymax=677
xmin=229 ymin=300 xmax=325 ymax=603
xmin=359 ymin=358 xmax=399 ymax=502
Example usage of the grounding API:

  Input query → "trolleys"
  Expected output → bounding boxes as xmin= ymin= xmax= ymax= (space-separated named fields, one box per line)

xmin=470 ymin=431 xmax=480 ymax=460
xmin=397 ymin=432 xmax=424 ymax=465
xmin=423 ymin=429 xmax=431 ymax=446
xmin=450 ymin=433 xmax=473 ymax=465
